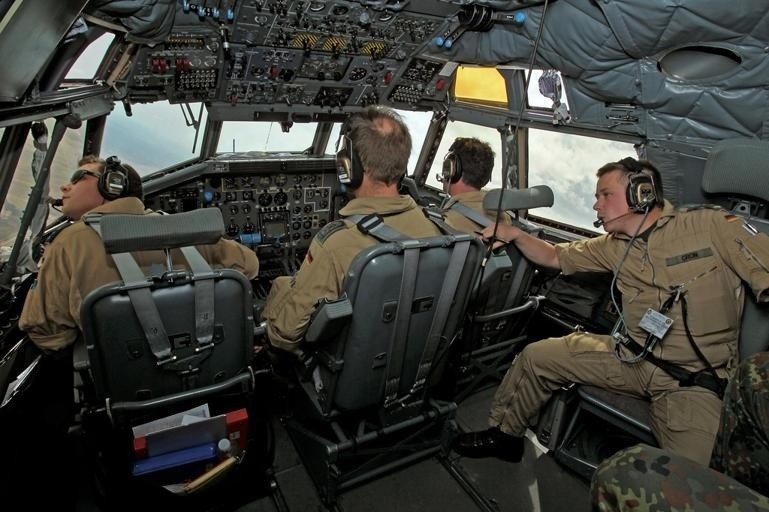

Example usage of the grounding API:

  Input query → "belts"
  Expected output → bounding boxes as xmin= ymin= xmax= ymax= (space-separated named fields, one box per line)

xmin=620 ymin=334 xmax=728 ymax=393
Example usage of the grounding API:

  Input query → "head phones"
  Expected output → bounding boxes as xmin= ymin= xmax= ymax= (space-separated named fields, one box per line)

xmin=335 ymin=119 xmax=363 ymax=191
xmin=620 ymin=156 xmax=659 ymax=215
xmin=97 ymin=155 xmax=129 ymax=202
xmin=442 ymin=138 xmax=470 ymax=183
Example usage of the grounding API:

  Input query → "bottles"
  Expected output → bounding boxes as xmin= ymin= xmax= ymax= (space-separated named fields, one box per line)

xmin=216 ymin=437 xmax=239 ymax=460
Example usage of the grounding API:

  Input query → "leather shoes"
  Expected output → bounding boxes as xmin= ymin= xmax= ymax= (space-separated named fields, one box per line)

xmin=454 ymin=427 xmax=524 ymax=463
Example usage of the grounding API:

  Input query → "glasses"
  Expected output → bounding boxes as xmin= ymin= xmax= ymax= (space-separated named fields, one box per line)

xmin=71 ymin=169 xmax=101 ymax=184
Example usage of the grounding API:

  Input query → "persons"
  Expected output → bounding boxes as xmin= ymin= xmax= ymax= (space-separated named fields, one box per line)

xmin=450 ymin=156 xmax=769 ymax=468
xmin=590 ymin=348 xmax=769 ymax=510
xmin=436 ymin=133 xmax=512 ymax=236
xmin=17 ymin=156 xmax=261 ymax=353
xmin=262 ymin=106 xmax=442 ymax=354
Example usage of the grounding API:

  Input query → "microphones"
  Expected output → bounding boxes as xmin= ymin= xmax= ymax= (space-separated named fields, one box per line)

xmin=436 ymin=172 xmax=449 ymax=181
xmin=593 ymin=193 xmax=655 ymax=229
xmin=52 ymin=199 xmax=63 ymax=213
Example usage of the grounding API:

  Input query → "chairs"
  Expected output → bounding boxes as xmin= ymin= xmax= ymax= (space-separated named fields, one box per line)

xmin=279 ymin=235 xmax=488 ymax=511
xmin=73 ymin=205 xmax=278 ymax=511
xmin=456 ymin=185 xmax=547 ymax=402
xmin=555 ymin=137 xmax=768 ymax=479
